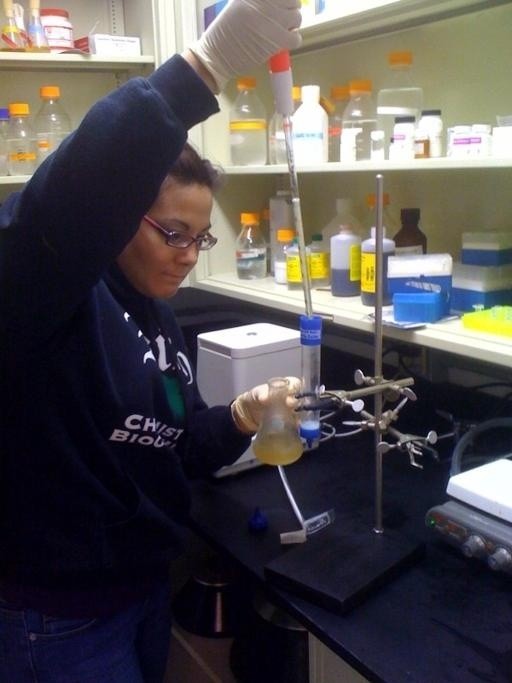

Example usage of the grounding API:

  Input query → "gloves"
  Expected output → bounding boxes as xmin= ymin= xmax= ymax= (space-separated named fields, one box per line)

xmin=187 ymin=0 xmax=304 ymax=96
xmin=233 ymin=375 xmax=304 ymax=435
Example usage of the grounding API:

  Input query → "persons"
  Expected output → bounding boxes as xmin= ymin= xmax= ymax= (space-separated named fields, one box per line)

xmin=0 ymin=0 xmax=303 ymax=679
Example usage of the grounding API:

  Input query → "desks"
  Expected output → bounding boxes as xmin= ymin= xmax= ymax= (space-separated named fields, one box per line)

xmin=122 ymin=331 xmax=512 ymax=682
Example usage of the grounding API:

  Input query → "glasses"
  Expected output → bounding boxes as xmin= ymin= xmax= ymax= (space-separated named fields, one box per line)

xmin=141 ymin=214 xmax=218 ymax=252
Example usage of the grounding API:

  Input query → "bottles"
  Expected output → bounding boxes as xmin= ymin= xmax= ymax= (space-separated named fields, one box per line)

xmin=296 ymin=313 xmax=327 ymax=438
xmin=0 ymin=85 xmax=69 ymax=177
xmin=252 ymin=378 xmax=303 ymax=467
xmin=233 ymin=192 xmax=427 ymax=306
xmin=0 ymin=0 xmax=50 ymax=55
xmin=228 ymin=43 xmax=489 ymax=169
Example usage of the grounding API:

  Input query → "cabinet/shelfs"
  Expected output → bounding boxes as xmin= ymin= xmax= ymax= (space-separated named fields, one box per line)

xmin=1 ymin=0 xmax=159 ymax=230
xmin=159 ymin=0 xmax=512 ymax=374
